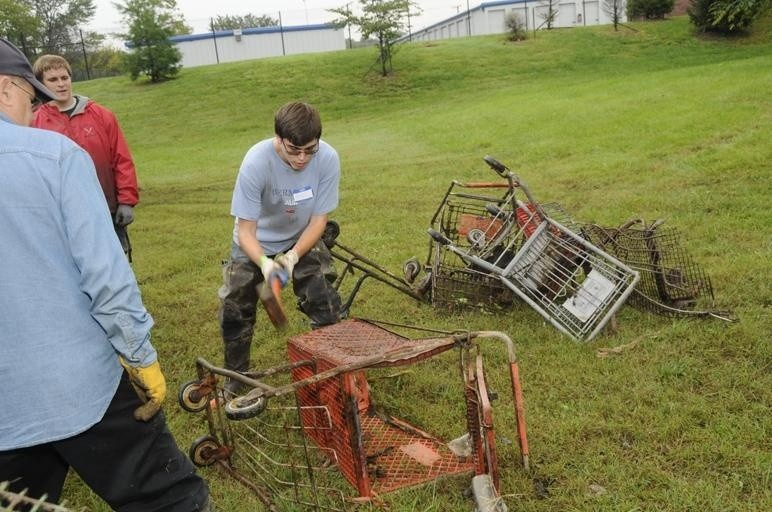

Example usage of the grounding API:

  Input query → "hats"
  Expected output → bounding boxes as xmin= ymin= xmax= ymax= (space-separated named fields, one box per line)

xmin=0 ymin=38 xmax=59 ymax=104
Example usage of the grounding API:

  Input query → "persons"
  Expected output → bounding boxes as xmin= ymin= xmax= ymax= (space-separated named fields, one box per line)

xmin=27 ymin=54 xmax=139 ymax=267
xmin=209 ymin=101 xmax=347 ymax=409
xmin=0 ymin=37 xmax=217 ymax=512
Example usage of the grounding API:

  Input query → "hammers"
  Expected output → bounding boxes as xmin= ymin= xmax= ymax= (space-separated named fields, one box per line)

xmin=255 ymin=277 xmax=287 ymax=329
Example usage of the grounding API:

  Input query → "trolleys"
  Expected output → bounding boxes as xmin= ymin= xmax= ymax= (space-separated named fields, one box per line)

xmin=318 ymin=215 xmax=433 ymax=319
xmin=179 ymin=316 xmax=531 ymax=512
xmin=424 ymin=154 xmax=641 ymax=346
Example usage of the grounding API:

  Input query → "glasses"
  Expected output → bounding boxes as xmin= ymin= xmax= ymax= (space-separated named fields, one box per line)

xmin=11 ymin=82 xmax=42 ymax=112
xmin=282 ymin=140 xmax=319 ymax=155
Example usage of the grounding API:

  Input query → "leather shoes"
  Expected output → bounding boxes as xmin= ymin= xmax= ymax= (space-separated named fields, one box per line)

xmin=211 ymin=388 xmax=242 ymax=407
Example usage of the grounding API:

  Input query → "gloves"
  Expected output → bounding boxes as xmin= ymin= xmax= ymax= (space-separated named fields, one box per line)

xmin=116 ymin=205 xmax=134 ymax=227
xmin=260 ymin=251 xmax=299 ymax=288
xmin=120 ymin=356 xmax=166 ymax=422
